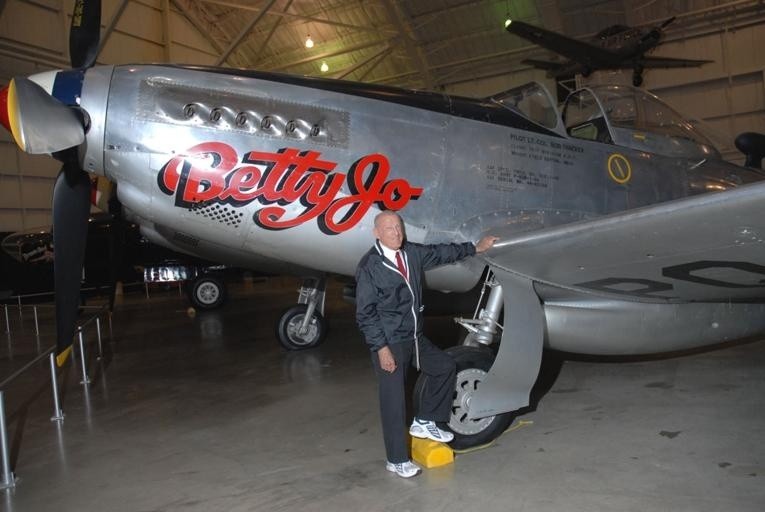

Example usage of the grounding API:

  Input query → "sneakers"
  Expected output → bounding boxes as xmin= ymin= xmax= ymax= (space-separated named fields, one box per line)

xmin=409 ymin=417 xmax=454 ymax=443
xmin=386 ymin=460 xmax=422 ymax=478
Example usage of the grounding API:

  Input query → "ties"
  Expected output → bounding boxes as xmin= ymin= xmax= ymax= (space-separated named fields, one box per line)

xmin=396 ymin=252 xmax=407 ymax=279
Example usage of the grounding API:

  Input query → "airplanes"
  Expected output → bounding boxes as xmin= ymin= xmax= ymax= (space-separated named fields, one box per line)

xmin=501 ymin=12 xmax=715 ymax=86
xmin=0 ymin=206 xmax=253 ymax=313
xmin=1 ymin=0 xmax=765 ymax=456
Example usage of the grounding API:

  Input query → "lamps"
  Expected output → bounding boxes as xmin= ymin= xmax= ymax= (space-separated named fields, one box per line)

xmin=304 ymin=21 xmax=314 ymax=48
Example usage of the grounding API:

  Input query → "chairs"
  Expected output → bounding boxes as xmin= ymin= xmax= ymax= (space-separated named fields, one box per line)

xmin=568 ymin=121 xmax=599 ymax=141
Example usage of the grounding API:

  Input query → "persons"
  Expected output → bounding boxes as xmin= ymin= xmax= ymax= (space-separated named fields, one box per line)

xmin=352 ymin=211 xmax=502 ymax=479
